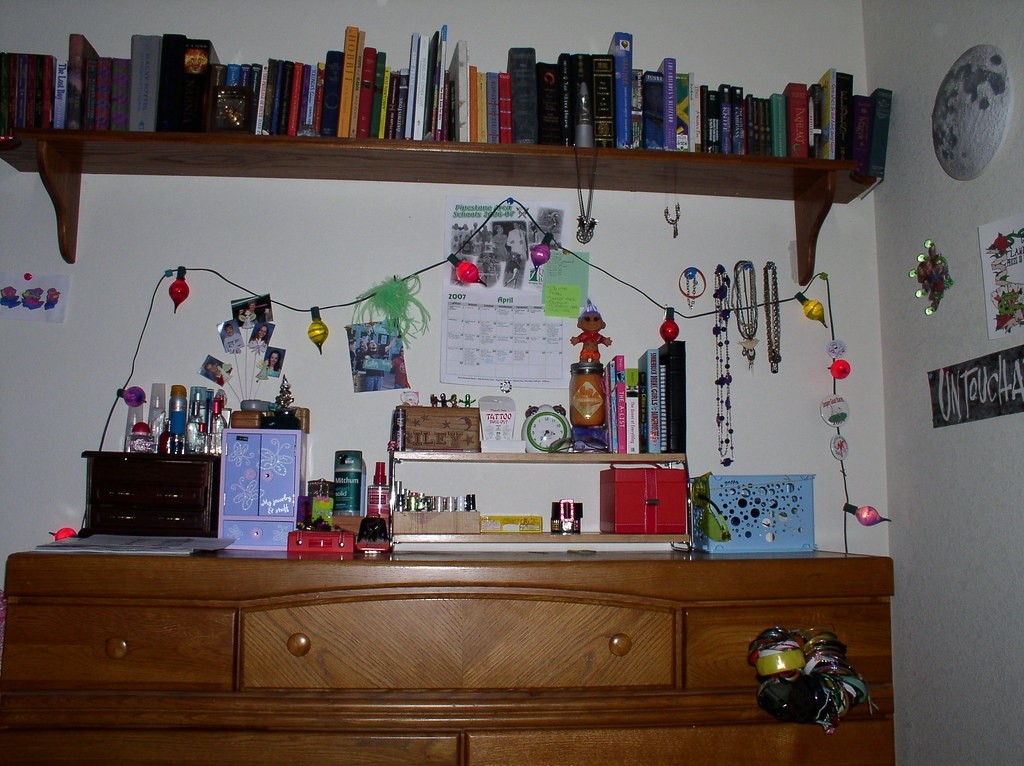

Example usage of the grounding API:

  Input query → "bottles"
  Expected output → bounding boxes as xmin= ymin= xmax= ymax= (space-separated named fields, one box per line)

xmin=129 ymin=392 xmax=227 ymax=455
xmin=367 ymin=461 xmax=389 ymax=544
xmin=569 ymin=362 xmax=605 ymax=427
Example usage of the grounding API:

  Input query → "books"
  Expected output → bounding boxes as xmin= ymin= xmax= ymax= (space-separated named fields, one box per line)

xmin=0 ymin=24 xmax=893 ymax=200
xmin=605 ymin=341 xmax=687 ymax=455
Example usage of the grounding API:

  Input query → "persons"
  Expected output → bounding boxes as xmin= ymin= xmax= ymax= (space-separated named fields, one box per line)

xmin=250 ymin=324 xmax=268 ymax=350
xmin=264 ymin=350 xmax=281 ymax=377
xmin=570 ymin=307 xmax=612 ymax=363
xmin=238 ymin=301 xmax=273 ymax=327
xmin=205 ymin=363 xmax=232 ymax=385
xmin=223 ymin=324 xmax=244 ymax=352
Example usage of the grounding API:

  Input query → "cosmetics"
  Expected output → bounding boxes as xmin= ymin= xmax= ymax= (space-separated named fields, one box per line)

xmin=395 ymin=481 xmax=477 ymax=513
xmin=331 ymin=448 xmax=391 ymax=536
xmin=125 ymin=383 xmax=230 ymax=455
xmin=660 ymin=307 xmax=679 ymax=342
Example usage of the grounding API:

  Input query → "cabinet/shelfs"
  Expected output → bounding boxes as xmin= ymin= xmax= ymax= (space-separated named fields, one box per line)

xmin=0 ymin=546 xmax=896 ymax=766
xmin=388 ymin=449 xmax=688 ymax=554
xmin=76 ymin=450 xmax=220 ymax=539
xmin=217 ymin=428 xmax=306 ymax=551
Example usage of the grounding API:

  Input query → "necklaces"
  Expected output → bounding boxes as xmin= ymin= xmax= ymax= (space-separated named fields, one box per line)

xmin=714 ymin=260 xmax=781 ymax=465
xmin=573 ymin=145 xmax=598 ymax=244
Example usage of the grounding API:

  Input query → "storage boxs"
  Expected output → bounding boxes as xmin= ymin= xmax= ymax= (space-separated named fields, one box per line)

xmin=392 ymin=510 xmax=480 ymax=534
xmin=480 ymin=516 xmax=543 ymax=534
xmin=689 ymin=471 xmax=817 ymax=554
xmin=396 ymin=405 xmax=479 ymax=450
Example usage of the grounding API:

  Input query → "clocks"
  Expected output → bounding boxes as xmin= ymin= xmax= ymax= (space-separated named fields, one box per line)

xmin=521 ymin=403 xmax=573 ymax=453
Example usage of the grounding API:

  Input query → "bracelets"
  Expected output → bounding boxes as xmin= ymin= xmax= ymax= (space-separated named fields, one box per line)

xmin=751 ymin=625 xmax=868 ymax=730
xmin=678 ymin=267 xmax=706 ymax=309
